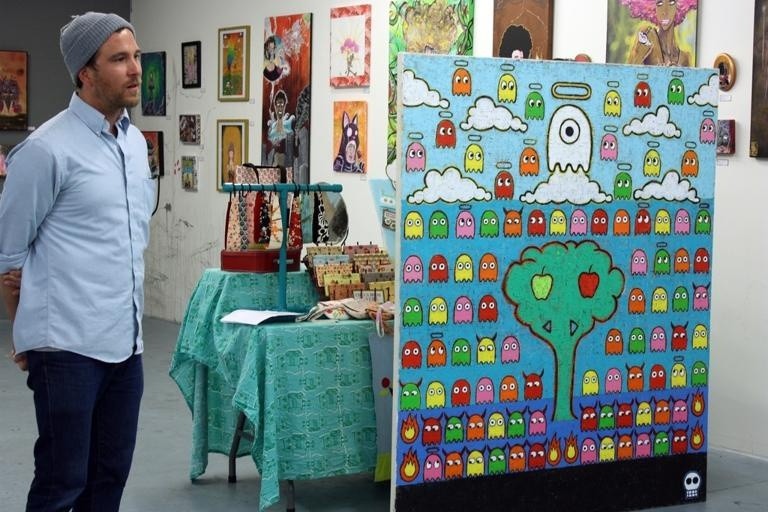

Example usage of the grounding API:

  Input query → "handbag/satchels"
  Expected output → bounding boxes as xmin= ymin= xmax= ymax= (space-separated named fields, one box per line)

xmin=222 ymin=163 xmax=295 ymax=253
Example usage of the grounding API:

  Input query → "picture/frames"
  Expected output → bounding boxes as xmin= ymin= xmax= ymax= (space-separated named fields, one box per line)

xmin=182 ymin=41 xmax=201 ymax=89
xmin=218 ymin=25 xmax=250 ymax=102
xmin=216 ymin=118 xmax=249 ymax=191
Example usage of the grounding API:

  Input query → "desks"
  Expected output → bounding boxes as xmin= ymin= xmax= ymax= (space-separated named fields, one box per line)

xmin=167 ymin=265 xmax=395 ymax=512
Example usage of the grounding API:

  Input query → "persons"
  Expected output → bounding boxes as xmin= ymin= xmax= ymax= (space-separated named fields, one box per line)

xmin=223 ymin=142 xmax=238 ymax=183
xmin=0 ymin=9 xmax=155 ymax=511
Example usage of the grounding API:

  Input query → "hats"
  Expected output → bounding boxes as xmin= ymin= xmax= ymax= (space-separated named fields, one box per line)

xmin=57 ymin=9 xmax=137 ymax=91
